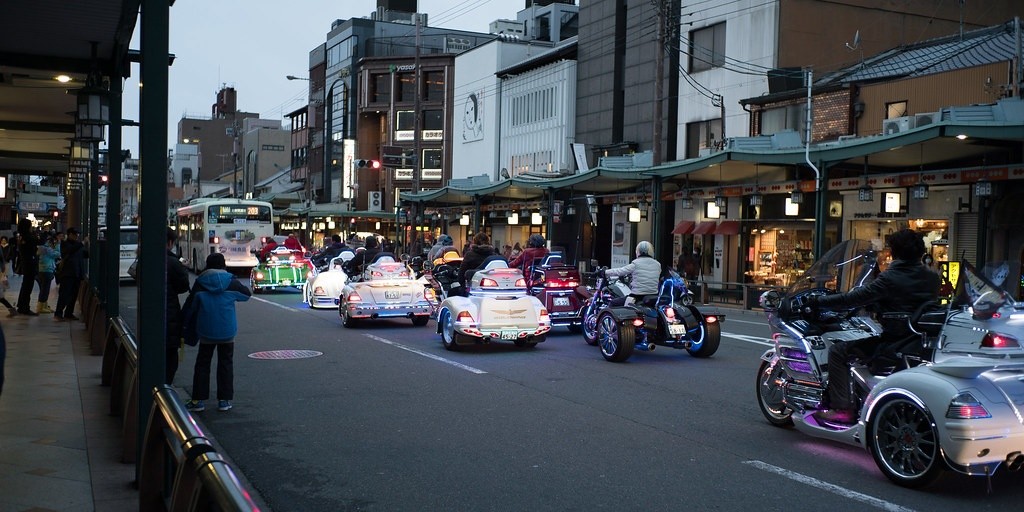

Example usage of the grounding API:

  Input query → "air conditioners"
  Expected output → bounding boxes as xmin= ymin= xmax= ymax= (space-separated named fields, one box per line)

xmin=882 ymin=116 xmax=914 ymax=136
xmin=914 ymin=112 xmax=939 ymax=128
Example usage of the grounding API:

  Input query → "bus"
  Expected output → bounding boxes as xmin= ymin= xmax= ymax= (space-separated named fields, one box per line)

xmin=176 ymin=197 xmax=274 ymax=275
xmin=99 ymin=226 xmax=138 ymax=284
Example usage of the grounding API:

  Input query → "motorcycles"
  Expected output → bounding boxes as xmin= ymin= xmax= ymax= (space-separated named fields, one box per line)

xmin=302 ymin=245 xmax=464 ymax=327
xmin=436 ymin=251 xmax=593 ymax=352
xmin=250 ymin=235 xmax=319 ymax=293
xmin=583 ymin=265 xmax=726 ymax=364
xmin=756 ymin=239 xmax=1024 ymax=491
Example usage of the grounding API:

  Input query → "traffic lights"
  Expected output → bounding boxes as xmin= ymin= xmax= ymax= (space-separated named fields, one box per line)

xmin=354 ymin=160 xmax=381 ymax=169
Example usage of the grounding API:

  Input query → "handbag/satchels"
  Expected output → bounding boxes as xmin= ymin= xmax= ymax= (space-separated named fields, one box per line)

xmin=55 ymin=264 xmax=63 ymax=285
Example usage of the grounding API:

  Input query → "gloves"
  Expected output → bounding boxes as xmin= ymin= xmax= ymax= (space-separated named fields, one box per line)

xmin=800 ymin=296 xmax=817 ymax=313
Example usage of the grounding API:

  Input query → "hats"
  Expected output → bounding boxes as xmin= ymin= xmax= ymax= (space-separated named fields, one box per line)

xmin=885 ymin=227 xmax=926 ymax=261
xmin=67 ymin=228 xmax=81 ymax=234
xmin=442 ymin=236 xmax=453 ymax=246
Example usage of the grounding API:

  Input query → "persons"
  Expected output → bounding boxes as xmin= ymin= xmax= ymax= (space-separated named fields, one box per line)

xmin=0 ymin=214 xmax=252 ymax=412
xmin=260 ymin=230 xmax=662 ymax=309
xmin=678 ymin=227 xmax=946 ymax=423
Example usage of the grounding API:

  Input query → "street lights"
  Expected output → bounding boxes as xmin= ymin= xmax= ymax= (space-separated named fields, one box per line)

xmin=288 ymin=75 xmax=326 ymax=205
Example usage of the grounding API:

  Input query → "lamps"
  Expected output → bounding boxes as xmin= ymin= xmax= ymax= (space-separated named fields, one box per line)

xmin=626 ymin=207 xmax=648 ymax=223
xmin=703 ymin=201 xmax=720 ymax=219
xmin=880 ymin=192 xmax=901 ymax=218
xmin=286 ymin=75 xmax=312 ymax=81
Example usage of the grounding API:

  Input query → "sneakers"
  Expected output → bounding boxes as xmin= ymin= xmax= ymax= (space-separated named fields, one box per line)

xmin=186 ymin=400 xmax=204 ymax=412
xmin=219 ymin=401 xmax=231 ymax=410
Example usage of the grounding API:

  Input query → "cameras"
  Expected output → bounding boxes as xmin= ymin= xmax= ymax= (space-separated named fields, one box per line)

xmin=179 ymin=257 xmax=186 ymax=262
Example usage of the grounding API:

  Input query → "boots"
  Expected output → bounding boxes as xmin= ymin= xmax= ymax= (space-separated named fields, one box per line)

xmin=37 ymin=301 xmax=54 ymax=313
xmin=6 ymin=307 xmax=17 ymax=318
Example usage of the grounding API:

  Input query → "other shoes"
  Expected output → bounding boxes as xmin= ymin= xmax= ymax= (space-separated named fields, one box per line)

xmin=817 ymin=410 xmax=856 ymax=422
xmin=17 ymin=308 xmax=37 ymax=316
xmin=65 ymin=314 xmax=79 ymax=320
xmin=54 ymin=313 xmax=61 ymax=318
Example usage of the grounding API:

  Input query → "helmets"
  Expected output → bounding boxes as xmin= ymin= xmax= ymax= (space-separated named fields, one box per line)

xmin=636 ymin=240 xmax=654 ymax=258
xmin=530 ymin=235 xmax=546 ymax=246
